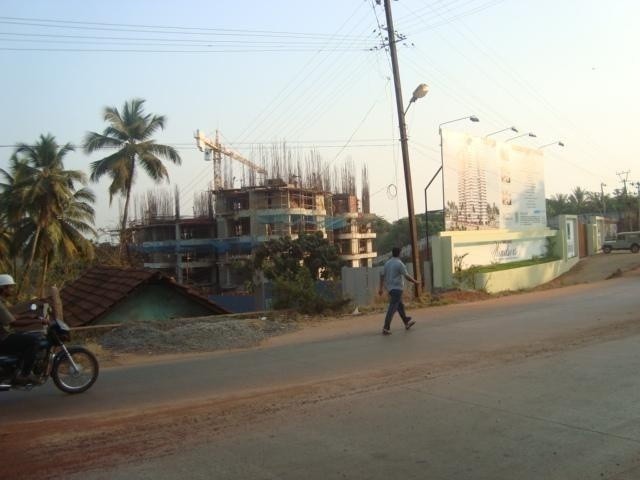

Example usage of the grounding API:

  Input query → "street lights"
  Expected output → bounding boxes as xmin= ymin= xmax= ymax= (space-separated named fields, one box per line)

xmin=438 ymin=115 xmax=564 ymax=232
xmin=382 ymin=0 xmax=428 ymax=308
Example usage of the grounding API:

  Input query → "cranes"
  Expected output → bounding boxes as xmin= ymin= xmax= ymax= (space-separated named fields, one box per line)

xmin=195 ymin=129 xmax=269 ymax=190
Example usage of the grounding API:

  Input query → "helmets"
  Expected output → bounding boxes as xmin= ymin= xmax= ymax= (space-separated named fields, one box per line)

xmin=0 ymin=274 xmax=16 ymax=285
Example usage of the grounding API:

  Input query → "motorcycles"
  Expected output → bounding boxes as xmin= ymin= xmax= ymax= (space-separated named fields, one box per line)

xmin=1 ymin=303 xmax=99 ymax=394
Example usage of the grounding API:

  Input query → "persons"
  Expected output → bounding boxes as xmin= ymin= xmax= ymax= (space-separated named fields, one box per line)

xmin=0 ymin=273 xmax=56 ymax=391
xmin=378 ymin=246 xmax=422 ymax=335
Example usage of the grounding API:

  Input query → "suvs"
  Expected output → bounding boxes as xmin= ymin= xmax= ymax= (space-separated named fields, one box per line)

xmin=602 ymin=231 xmax=640 ymax=253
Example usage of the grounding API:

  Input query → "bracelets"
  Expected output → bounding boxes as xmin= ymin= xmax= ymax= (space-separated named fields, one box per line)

xmin=40 ymin=318 xmax=43 ymax=324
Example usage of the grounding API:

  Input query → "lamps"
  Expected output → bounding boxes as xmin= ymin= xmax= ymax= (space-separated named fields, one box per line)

xmin=439 ymin=115 xmax=480 ymax=134
xmin=539 ymin=141 xmax=564 ymax=149
xmin=487 ymin=126 xmax=518 ymax=137
xmin=506 ymin=133 xmax=536 ymax=142
